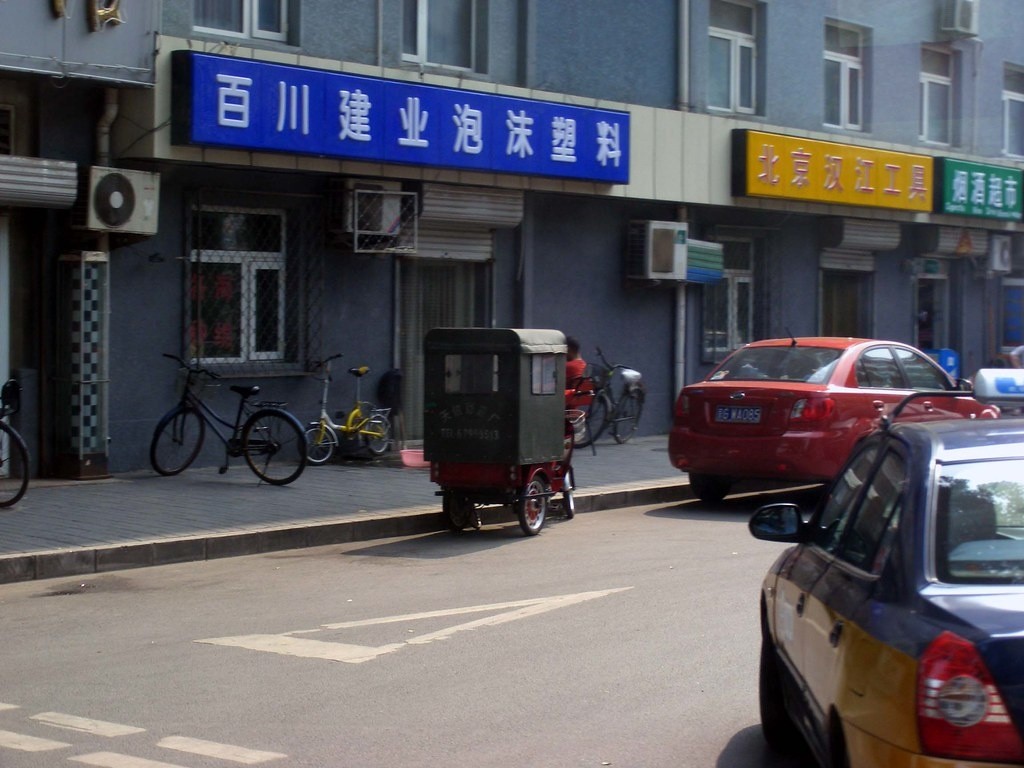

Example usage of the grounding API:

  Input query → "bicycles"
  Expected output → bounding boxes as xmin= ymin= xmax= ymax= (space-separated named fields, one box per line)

xmin=572 ymin=346 xmax=646 ymax=456
xmin=295 ymin=350 xmax=393 ymax=466
xmin=149 ymin=353 xmax=309 ymax=488
xmin=0 ymin=379 xmax=32 ymax=507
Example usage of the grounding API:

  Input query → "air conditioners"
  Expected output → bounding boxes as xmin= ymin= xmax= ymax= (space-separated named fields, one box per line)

xmin=329 ymin=178 xmax=402 ymax=236
xmin=625 ymin=220 xmax=689 ymax=280
xmin=71 ymin=165 xmax=161 ymax=236
xmin=979 ymin=234 xmax=1012 ymax=272
xmin=940 ymin=0 xmax=979 ymax=34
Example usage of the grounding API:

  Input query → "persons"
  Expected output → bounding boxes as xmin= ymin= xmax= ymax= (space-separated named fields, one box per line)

xmin=562 ymin=334 xmax=594 ymax=421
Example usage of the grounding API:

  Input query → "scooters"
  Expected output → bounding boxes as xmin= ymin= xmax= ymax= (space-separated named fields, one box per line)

xmin=422 ymin=327 xmax=595 ymax=536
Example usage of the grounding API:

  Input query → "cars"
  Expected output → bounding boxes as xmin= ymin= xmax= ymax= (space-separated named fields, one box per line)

xmin=668 ymin=333 xmax=1003 ymax=505
xmin=748 ymin=368 xmax=1024 ymax=768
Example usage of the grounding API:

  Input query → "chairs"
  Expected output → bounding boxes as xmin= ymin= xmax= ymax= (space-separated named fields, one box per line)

xmin=937 ymin=489 xmax=998 ymax=541
